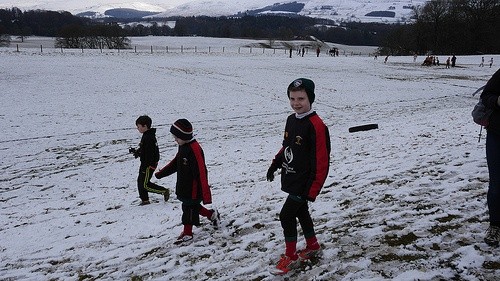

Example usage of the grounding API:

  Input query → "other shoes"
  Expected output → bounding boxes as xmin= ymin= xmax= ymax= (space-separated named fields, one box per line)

xmin=163 ymin=188 xmax=170 ymax=202
xmin=138 ymin=201 xmax=150 ymax=206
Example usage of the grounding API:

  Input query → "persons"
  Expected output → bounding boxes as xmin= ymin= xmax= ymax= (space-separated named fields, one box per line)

xmin=488 ymin=58 xmax=493 ymax=68
xmin=384 ymin=55 xmax=389 ymax=64
xmin=373 ymin=48 xmax=380 ymax=60
xmin=316 ymin=47 xmax=320 ymax=57
xmin=446 ymin=55 xmax=456 ymax=69
xmin=155 ymin=119 xmax=220 ymax=247
xmin=129 ymin=115 xmax=170 ymax=206
xmin=266 ymin=78 xmax=331 ymax=276
xmin=289 ymin=46 xmax=305 ymax=58
xmin=421 ymin=54 xmax=440 ymax=67
xmin=329 ymin=48 xmax=339 ymax=57
xmin=479 ymin=69 xmax=500 ymax=247
xmin=479 ymin=57 xmax=484 ymax=67
xmin=413 ymin=53 xmax=417 ymax=62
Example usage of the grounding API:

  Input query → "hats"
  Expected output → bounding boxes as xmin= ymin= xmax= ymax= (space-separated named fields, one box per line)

xmin=286 ymin=77 xmax=316 ymax=106
xmin=170 ymin=119 xmax=193 ymax=141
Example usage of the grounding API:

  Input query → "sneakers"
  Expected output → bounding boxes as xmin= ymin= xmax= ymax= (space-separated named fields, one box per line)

xmin=207 ymin=208 xmax=221 ymax=229
xmin=484 ymin=224 xmax=500 ymax=246
xmin=297 ymin=246 xmax=322 ymax=260
xmin=173 ymin=233 xmax=193 ymax=245
xmin=268 ymin=254 xmax=302 ymax=275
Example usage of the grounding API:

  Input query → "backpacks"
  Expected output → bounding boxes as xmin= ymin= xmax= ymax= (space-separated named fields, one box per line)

xmin=473 ymin=83 xmax=487 ymax=127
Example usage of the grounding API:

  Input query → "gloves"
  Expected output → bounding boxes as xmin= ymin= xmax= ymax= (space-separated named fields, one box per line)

xmin=266 ymin=168 xmax=275 ymax=183
xmin=154 ymin=172 xmax=163 ymax=180
